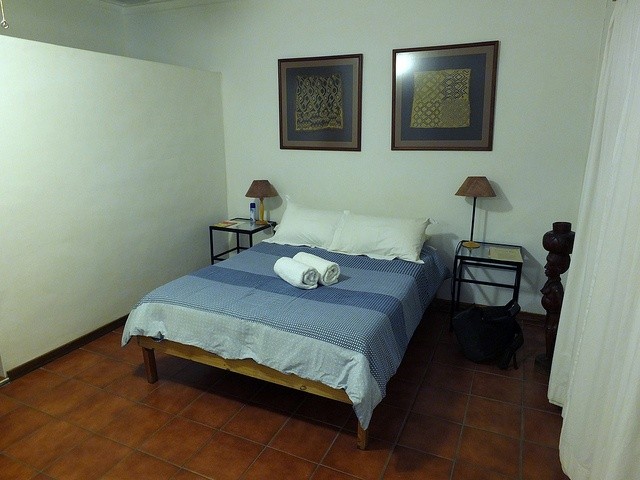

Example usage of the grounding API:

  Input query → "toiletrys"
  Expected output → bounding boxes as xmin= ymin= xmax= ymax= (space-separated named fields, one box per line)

xmin=248 ymin=203 xmax=257 ymax=232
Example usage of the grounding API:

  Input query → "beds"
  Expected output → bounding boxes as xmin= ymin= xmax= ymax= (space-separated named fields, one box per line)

xmin=121 ymin=194 xmax=451 ymax=451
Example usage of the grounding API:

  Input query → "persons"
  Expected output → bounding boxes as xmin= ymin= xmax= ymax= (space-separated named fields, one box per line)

xmin=535 ymin=254 xmax=570 ymax=367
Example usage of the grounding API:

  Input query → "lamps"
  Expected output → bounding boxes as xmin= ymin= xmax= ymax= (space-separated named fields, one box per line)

xmin=455 ymin=176 xmax=497 ymax=248
xmin=244 ymin=180 xmax=278 ymax=225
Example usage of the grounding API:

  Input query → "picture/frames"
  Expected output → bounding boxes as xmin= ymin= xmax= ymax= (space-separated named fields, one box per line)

xmin=391 ymin=40 xmax=499 ymax=151
xmin=277 ymin=54 xmax=362 ymax=152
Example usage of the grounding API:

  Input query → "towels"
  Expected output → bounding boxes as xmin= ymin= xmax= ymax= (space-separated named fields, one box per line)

xmin=293 ymin=251 xmax=340 ymax=286
xmin=274 ymin=256 xmax=319 ymax=290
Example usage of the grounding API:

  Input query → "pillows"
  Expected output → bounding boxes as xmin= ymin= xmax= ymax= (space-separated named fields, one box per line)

xmin=328 ymin=210 xmax=434 ymax=265
xmin=261 ymin=195 xmax=341 ymax=251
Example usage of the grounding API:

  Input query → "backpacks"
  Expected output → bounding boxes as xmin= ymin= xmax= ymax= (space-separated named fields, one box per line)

xmin=452 ymin=300 xmax=524 ymax=370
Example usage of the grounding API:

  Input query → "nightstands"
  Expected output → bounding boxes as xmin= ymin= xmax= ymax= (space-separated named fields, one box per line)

xmin=209 ymin=218 xmax=276 ymax=265
xmin=452 ymin=240 xmax=524 ymax=303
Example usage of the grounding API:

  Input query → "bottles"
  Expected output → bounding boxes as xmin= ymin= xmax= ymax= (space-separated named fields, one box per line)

xmin=249 ymin=202 xmax=256 ymax=223
